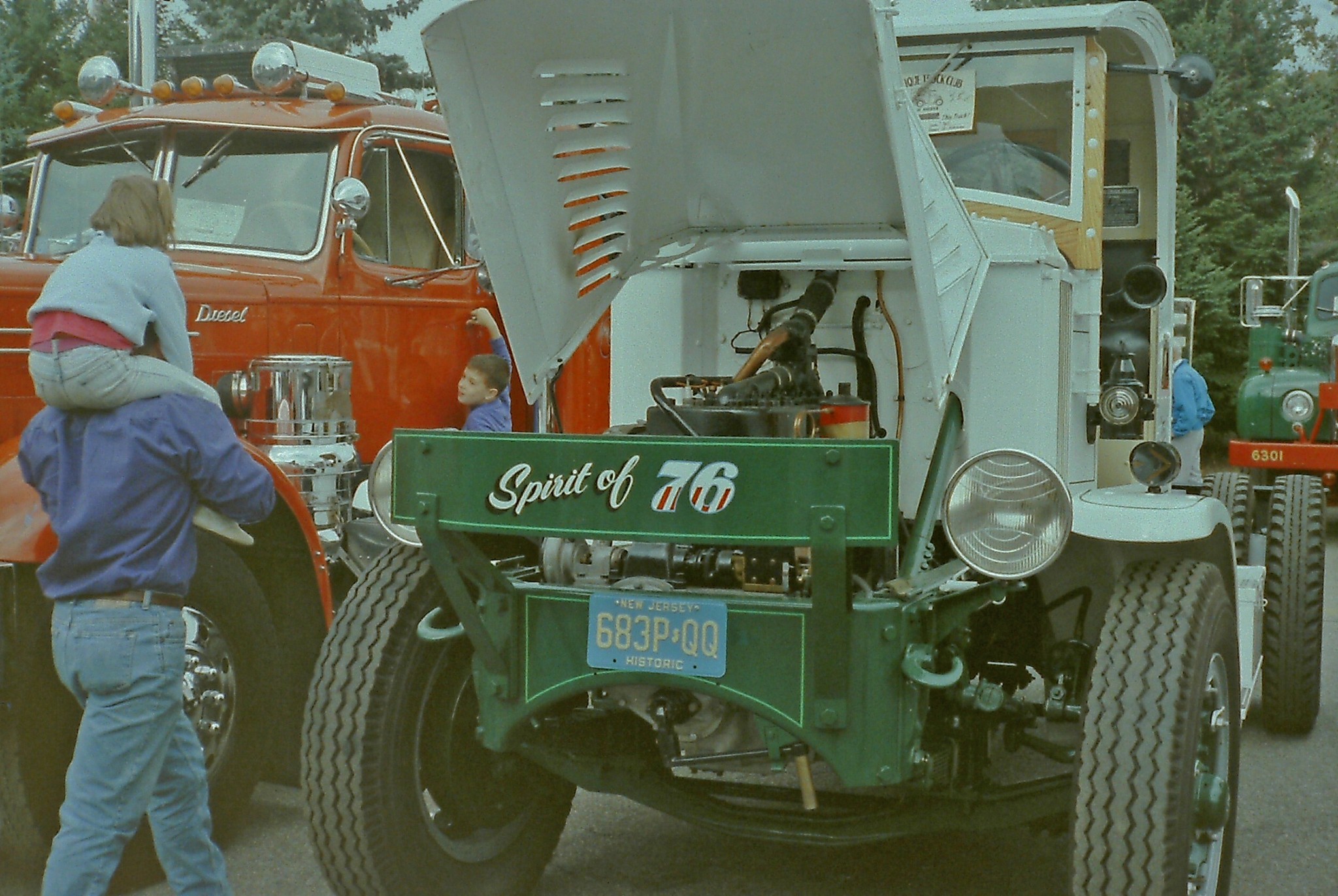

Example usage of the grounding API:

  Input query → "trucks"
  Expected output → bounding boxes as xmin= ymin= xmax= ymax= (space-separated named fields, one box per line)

xmin=1226 ymin=186 xmax=1338 ymax=505
xmin=1 ymin=39 xmax=614 ymax=896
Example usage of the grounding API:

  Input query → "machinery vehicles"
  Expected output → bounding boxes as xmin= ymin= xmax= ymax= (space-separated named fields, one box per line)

xmin=292 ymin=1 xmax=1330 ymax=896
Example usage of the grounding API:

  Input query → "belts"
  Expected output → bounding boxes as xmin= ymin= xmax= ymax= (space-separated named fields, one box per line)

xmin=65 ymin=591 xmax=184 ymax=609
xmin=31 ymin=338 xmax=91 ymax=353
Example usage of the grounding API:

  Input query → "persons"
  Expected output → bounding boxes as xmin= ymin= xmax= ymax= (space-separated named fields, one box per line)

xmin=25 ymin=176 xmax=260 ymax=547
xmin=0 ymin=318 xmax=278 ymax=896
xmin=1171 ymin=344 xmax=1216 ymax=486
xmin=456 ymin=307 xmax=518 ymax=433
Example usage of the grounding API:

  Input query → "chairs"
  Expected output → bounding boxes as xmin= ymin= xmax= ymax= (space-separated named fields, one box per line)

xmin=231 ymin=151 xmax=440 ymax=273
